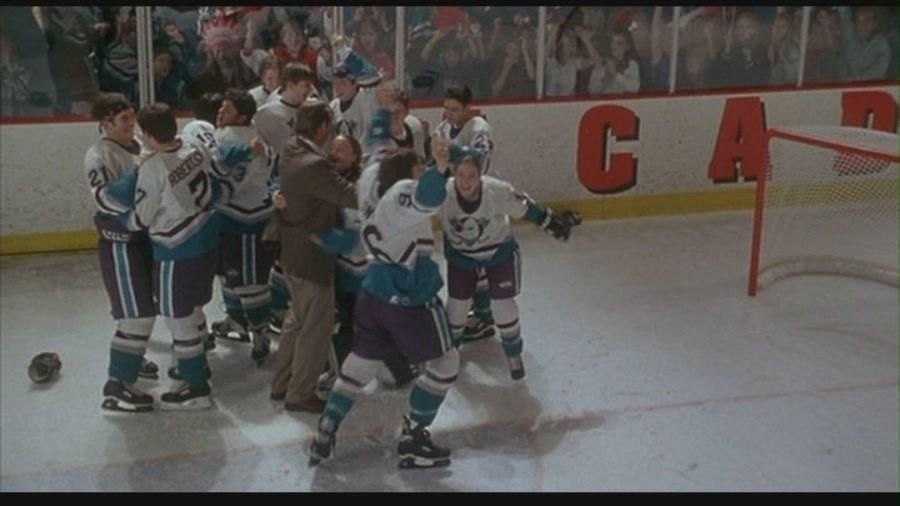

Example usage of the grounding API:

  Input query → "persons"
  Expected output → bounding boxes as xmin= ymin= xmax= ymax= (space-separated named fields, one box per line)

xmin=308 ymin=136 xmax=460 ymax=467
xmin=0 ymin=0 xmax=898 ymax=120
xmin=83 ymin=53 xmax=583 ymax=410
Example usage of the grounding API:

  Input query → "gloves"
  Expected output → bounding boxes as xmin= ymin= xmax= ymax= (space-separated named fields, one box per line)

xmin=27 ymin=353 xmax=62 ymax=384
xmin=538 ymin=208 xmax=570 ymax=241
